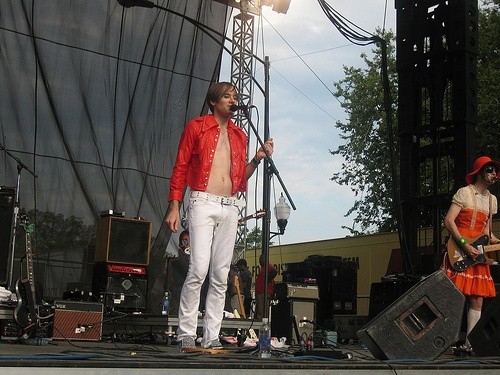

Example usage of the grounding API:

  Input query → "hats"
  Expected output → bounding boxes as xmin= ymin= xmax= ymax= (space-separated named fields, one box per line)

xmin=465 ymin=156 xmax=500 ymax=185
xmin=234 ymin=259 xmax=246 ymax=266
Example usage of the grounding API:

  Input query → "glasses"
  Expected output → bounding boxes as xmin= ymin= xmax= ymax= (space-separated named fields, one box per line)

xmin=484 ymin=166 xmax=499 ymax=173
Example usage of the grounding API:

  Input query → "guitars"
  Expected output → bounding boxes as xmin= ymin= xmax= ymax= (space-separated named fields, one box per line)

xmin=234 ymin=274 xmax=258 ymax=339
xmin=448 ymin=233 xmax=500 ymax=272
xmin=179 ymin=208 xmax=267 ymax=256
xmin=13 ymin=213 xmax=43 ymax=331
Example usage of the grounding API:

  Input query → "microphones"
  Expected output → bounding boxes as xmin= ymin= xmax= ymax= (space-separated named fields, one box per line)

xmin=230 ymin=104 xmax=254 ymax=111
xmin=77 ymin=324 xmax=92 ymax=328
xmin=300 ymin=320 xmax=309 ymax=323
xmin=494 ymin=176 xmax=500 ymax=181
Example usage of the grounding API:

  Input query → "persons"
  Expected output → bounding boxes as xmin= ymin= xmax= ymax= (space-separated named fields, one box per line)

xmin=237 ymin=259 xmax=252 ymax=320
xmin=255 ymin=254 xmax=277 ymax=322
xmin=178 ymin=228 xmax=191 ymax=254
xmin=164 ymin=82 xmax=273 ymax=348
xmin=440 ymin=155 xmax=500 ymax=352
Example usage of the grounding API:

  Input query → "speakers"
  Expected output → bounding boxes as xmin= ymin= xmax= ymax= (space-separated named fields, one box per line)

xmin=106 ymin=277 xmax=149 ymax=311
xmin=93 ymin=216 xmax=152 ymax=267
xmin=51 ymin=300 xmax=106 ymax=341
xmin=272 ymin=300 xmax=316 ymax=347
xmin=357 ymin=271 xmax=469 ymax=363
xmin=0 ymin=190 xmax=16 ymax=285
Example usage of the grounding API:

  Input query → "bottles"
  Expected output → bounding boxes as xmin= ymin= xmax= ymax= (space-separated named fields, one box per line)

xmin=258 ymin=317 xmax=270 ymax=359
xmin=161 ymin=291 xmax=170 ymax=314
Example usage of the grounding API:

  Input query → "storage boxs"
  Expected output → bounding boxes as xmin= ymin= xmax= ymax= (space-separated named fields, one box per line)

xmin=326 ymin=331 xmax=337 ymax=346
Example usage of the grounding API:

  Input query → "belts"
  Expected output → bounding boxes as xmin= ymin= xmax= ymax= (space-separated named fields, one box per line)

xmin=190 ymin=190 xmax=247 ymax=207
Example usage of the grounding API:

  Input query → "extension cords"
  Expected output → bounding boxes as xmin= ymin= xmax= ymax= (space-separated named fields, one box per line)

xmin=294 ymin=349 xmax=346 ymax=360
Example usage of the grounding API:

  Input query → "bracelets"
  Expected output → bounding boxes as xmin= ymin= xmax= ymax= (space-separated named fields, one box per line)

xmin=456 ymin=237 xmax=468 ymax=249
xmin=250 ymin=157 xmax=260 ymax=168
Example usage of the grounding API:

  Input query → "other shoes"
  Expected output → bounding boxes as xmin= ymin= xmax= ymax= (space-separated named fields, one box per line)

xmin=211 ymin=339 xmax=223 ymax=349
xmin=180 ymin=339 xmax=196 ymax=352
